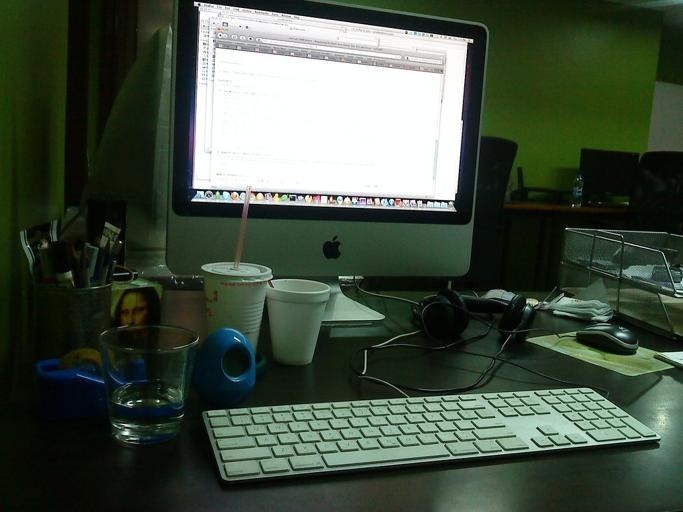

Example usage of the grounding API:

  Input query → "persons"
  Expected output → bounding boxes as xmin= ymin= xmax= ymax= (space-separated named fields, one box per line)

xmin=112 ymin=288 xmax=161 ymax=348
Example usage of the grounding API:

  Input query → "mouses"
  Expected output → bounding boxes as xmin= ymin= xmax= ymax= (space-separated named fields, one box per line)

xmin=575 ymin=321 xmax=643 ymax=355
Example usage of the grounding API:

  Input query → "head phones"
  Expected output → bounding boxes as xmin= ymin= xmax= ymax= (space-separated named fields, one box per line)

xmin=412 ymin=285 xmax=537 ymax=346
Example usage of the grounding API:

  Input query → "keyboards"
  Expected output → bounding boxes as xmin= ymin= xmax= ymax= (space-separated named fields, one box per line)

xmin=199 ymin=385 xmax=662 ymax=482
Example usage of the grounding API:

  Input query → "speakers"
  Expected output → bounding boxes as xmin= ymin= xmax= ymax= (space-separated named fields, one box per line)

xmin=201 ymin=326 xmax=258 ymax=405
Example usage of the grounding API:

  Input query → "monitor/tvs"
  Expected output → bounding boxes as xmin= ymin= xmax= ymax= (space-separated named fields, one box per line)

xmin=169 ymin=0 xmax=492 ymax=327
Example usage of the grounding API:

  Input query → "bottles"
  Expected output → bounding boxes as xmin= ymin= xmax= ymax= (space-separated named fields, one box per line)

xmin=572 ymin=169 xmax=585 ymax=209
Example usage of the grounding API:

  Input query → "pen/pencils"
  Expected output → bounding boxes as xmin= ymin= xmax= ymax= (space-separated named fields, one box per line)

xmin=85 ymin=260 xmax=90 ymax=337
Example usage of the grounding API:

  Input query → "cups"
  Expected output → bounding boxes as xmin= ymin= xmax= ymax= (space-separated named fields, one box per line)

xmin=35 ymin=279 xmax=112 ymax=363
xmin=201 ymin=260 xmax=273 ymax=366
xmin=266 ymin=277 xmax=332 ymax=369
xmin=99 ymin=323 xmax=200 ymax=450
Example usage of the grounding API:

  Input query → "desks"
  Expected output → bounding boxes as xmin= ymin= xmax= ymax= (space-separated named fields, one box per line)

xmin=501 ymin=199 xmax=631 ymax=289
xmin=0 ymin=276 xmax=682 ymax=512
